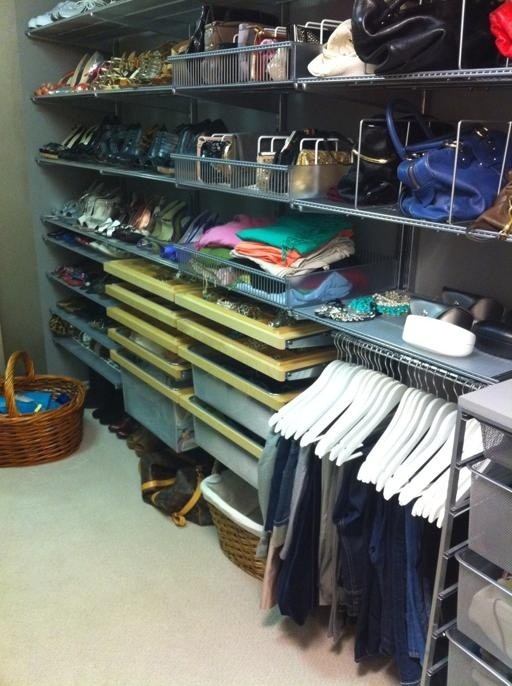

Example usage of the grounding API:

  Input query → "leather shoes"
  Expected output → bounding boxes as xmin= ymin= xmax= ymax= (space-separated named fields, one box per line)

xmin=93 ymin=402 xmax=158 ymax=456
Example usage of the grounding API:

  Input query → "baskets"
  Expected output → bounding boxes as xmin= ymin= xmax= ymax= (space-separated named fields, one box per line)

xmin=0 ymin=351 xmax=85 ymax=468
xmin=201 ymin=469 xmax=268 ymax=579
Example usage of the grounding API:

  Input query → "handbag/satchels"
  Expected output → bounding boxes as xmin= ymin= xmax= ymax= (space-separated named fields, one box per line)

xmin=141 ymin=454 xmax=212 ymax=526
